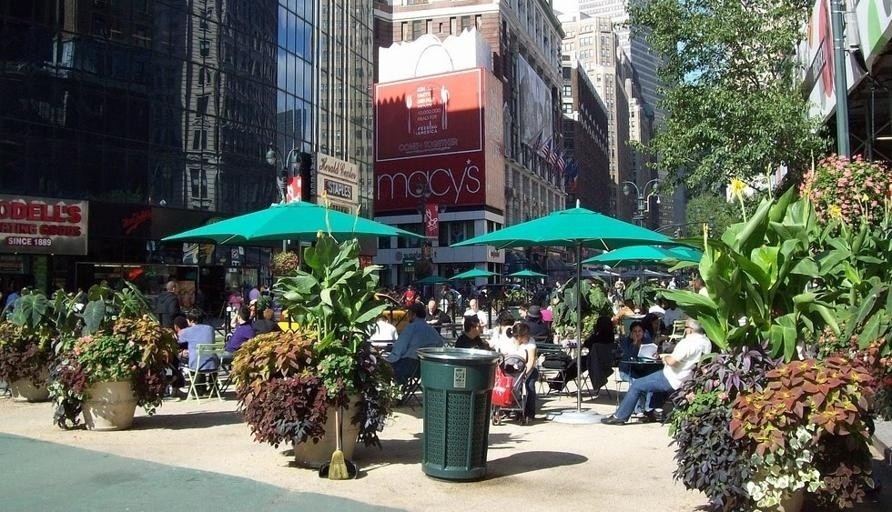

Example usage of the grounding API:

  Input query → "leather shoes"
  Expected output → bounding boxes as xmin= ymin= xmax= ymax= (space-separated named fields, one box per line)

xmin=647 ymin=410 xmax=664 ymax=422
xmin=601 ymin=415 xmax=625 ymax=425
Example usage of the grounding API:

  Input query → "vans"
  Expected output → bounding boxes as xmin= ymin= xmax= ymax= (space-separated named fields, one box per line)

xmin=473 ymin=283 xmax=525 ymax=304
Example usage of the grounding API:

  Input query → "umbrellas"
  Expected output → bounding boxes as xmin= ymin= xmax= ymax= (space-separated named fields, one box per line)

xmin=450 ymin=201 xmax=695 ymax=425
xmin=162 ymin=202 xmax=426 ymax=255
xmin=452 ymin=267 xmax=503 ymax=285
xmin=416 ymin=276 xmax=449 ymax=284
xmin=666 ymin=247 xmax=704 ymax=264
xmin=572 ymin=245 xmax=693 ymax=288
xmin=505 ymin=268 xmax=547 ymax=289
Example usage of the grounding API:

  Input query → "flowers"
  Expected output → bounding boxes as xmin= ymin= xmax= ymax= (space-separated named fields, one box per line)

xmin=663 ymin=153 xmax=892 ymax=512
xmin=0 ymin=286 xmax=73 ymax=385
xmin=229 ymin=235 xmax=399 ymax=446
xmin=47 ymin=277 xmax=185 ymax=431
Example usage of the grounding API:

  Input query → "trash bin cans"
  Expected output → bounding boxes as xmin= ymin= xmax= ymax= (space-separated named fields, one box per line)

xmin=416 ymin=347 xmax=501 ymax=482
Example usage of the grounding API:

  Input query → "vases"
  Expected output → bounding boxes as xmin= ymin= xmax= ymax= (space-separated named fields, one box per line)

xmin=290 ymin=392 xmax=368 ymax=471
xmin=74 ymin=370 xmax=140 ymax=433
xmin=14 ymin=363 xmax=57 ymax=402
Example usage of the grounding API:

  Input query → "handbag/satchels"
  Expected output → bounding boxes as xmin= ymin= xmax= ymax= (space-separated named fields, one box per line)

xmin=491 ymin=366 xmax=512 ymax=407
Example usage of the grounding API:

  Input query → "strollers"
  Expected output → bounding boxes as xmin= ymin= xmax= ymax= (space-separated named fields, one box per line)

xmin=490 ymin=349 xmax=529 ymax=427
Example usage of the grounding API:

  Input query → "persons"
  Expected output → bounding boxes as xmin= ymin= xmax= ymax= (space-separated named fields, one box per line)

xmin=455 ymin=283 xmax=553 ymax=423
xmin=601 ymin=299 xmax=711 ymax=424
xmin=363 ymin=284 xmax=448 ymax=376
xmin=157 ymin=281 xmax=278 ymax=397
xmin=549 ymin=315 xmax=614 ymax=390
xmin=3 ymin=281 xmax=88 ymax=311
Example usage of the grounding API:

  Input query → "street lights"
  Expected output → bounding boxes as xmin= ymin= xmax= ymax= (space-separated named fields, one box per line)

xmin=644 ymin=192 xmax=661 ymax=212
xmin=266 ymin=142 xmax=302 ymax=252
xmin=621 ymin=179 xmax=664 ymax=227
xmin=415 ymin=181 xmax=434 ymax=295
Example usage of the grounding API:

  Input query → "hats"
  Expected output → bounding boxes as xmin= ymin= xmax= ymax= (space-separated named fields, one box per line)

xmin=527 ymin=305 xmax=540 ymax=318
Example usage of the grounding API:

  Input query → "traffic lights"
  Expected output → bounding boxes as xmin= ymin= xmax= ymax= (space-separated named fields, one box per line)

xmin=674 ymin=230 xmax=682 ymax=239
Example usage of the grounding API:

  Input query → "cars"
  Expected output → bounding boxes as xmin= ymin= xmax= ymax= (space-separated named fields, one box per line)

xmin=374 ymin=292 xmax=410 ymax=333
